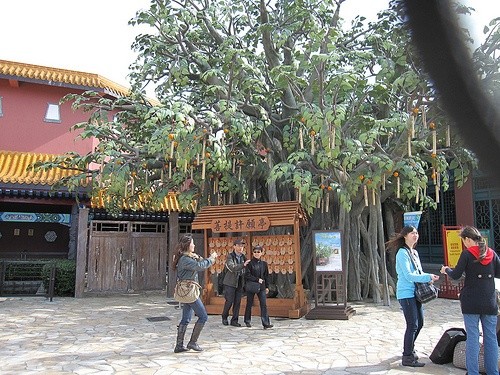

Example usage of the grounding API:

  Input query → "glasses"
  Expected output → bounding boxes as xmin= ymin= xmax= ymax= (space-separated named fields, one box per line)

xmin=254 ymin=251 xmax=260 ymax=253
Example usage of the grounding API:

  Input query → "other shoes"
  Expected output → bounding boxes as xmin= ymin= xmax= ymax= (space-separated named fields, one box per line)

xmin=402 ymin=347 xmax=419 ymax=360
xmin=402 ymin=355 xmax=425 ymax=367
xmin=264 ymin=325 xmax=273 ymax=329
xmin=231 ymin=319 xmax=241 ymax=327
xmin=222 ymin=313 xmax=228 ymax=325
xmin=246 ymin=322 xmax=252 ymax=327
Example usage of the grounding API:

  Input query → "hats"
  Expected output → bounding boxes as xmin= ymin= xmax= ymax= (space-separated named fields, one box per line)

xmin=233 ymin=240 xmax=245 ymax=244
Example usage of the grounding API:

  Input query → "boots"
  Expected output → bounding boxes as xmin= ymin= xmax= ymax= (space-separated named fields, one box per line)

xmin=187 ymin=322 xmax=205 ymax=351
xmin=174 ymin=323 xmax=189 ymax=352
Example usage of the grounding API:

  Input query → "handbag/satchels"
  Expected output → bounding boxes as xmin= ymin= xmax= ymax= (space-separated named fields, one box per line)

xmin=415 ymin=281 xmax=438 ymax=304
xmin=173 ymin=279 xmax=202 ymax=303
xmin=453 ymin=340 xmax=500 ymax=372
xmin=246 ymin=280 xmax=260 ymax=294
xmin=429 ymin=328 xmax=467 ymax=364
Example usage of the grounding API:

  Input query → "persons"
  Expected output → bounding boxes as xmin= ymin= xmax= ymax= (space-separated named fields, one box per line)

xmin=174 ymin=235 xmax=217 ymax=353
xmin=441 ymin=225 xmax=500 ymax=375
xmin=386 ymin=224 xmax=440 ymax=367
xmin=244 ymin=246 xmax=274 ymax=329
xmin=222 ymin=240 xmax=251 ymax=325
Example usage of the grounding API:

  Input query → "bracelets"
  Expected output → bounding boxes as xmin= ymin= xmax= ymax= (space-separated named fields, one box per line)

xmin=198 ymin=253 xmax=200 ymax=259
